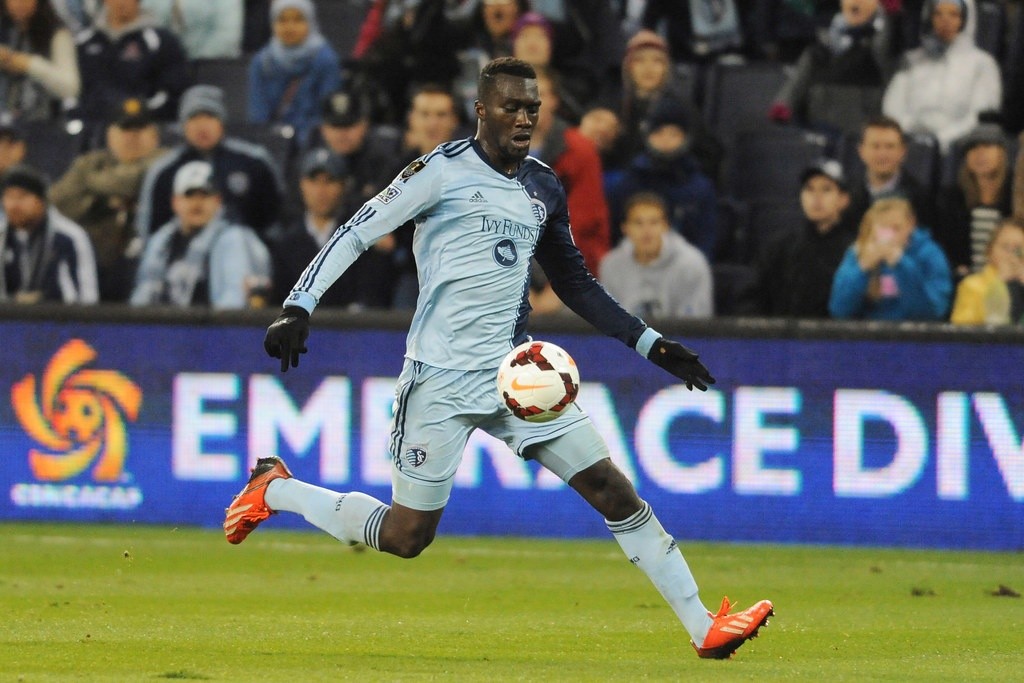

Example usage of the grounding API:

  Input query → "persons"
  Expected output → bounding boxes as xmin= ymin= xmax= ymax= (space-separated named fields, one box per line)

xmin=0 ymin=0 xmax=1024 ymax=326
xmin=224 ymin=56 xmax=775 ymax=660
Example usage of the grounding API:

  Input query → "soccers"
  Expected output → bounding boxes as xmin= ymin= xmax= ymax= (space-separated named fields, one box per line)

xmin=495 ymin=339 xmax=581 ymax=424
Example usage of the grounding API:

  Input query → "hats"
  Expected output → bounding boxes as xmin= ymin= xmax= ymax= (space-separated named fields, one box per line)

xmin=796 ymin=154 xmax=848 ymax=196
xmin=175 ymin=161 xmax=220 ymax=196
xmin=963 ymin=124 xmax=1009 ymax=154
xmin=320 ymin=93 xmax=367 ymax=127
xmin=626 ymin=32 xmax=668 ymax=57
xmin=302 ymin=149 xmax=344 ymax=176
xmin=182 ymin=85 xmax=224 ymax=120
xmin=1 ymin=163 xmax=51 ymax=200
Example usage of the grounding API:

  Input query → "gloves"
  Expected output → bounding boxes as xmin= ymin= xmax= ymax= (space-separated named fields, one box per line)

xmin=264 ymin=306 xmax=310 ymax=372
xmin=647 ymin=338 xmax=716 ymax=392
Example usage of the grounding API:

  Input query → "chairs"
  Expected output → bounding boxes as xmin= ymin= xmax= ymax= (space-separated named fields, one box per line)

xmin=14 ymin=0 xmax=1024 ymax=319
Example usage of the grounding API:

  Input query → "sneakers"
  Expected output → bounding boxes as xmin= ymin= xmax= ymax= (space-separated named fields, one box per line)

xmin=690 ymin=596 xmax=775 ymax=659
xmin=223 ymin=456 xmax=293 ymax=545
